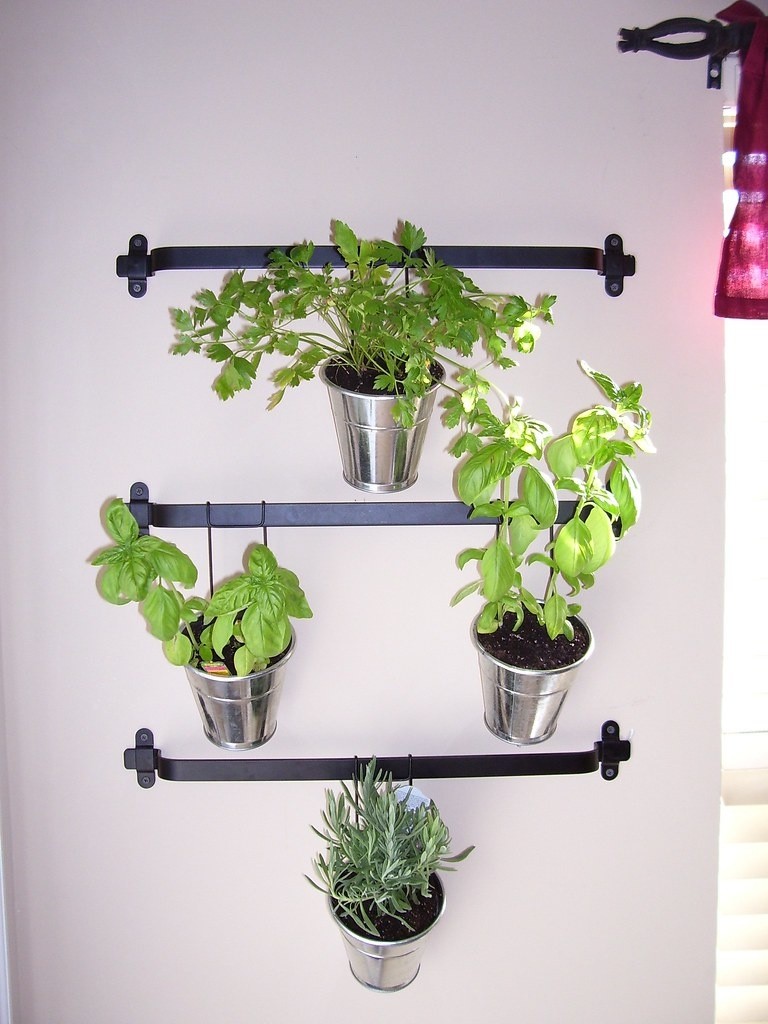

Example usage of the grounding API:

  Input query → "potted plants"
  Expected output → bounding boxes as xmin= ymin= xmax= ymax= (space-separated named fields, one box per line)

xmin=91 ymin=498 xmax=312 ymax=751
xmin=169 ymin=220 xmax=558 ymax=493
xmin=441 ymin=359 xmax=657 ymax=746
xmin=305 ymin=755 xmax=476 ymax=993
